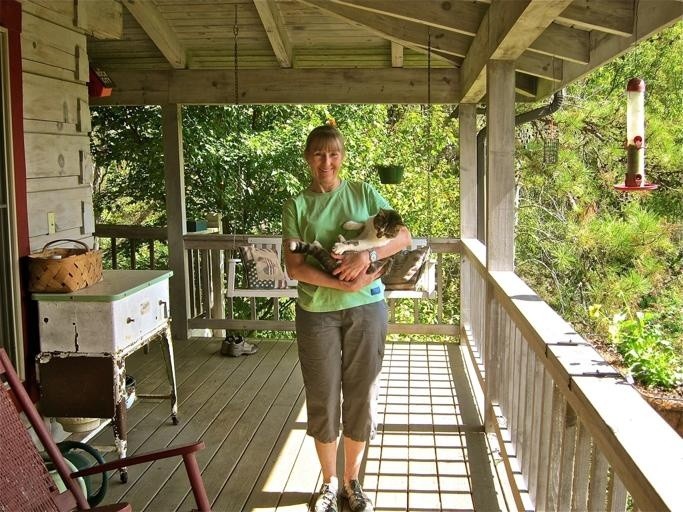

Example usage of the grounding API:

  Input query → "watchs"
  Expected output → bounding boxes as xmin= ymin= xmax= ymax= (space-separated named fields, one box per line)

xmin=363 ymin=248 xmax=379 ymax=266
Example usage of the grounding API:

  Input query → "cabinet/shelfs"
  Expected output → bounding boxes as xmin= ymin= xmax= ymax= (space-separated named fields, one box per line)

xmin=31 ymin=269 xmax=180 ymax=485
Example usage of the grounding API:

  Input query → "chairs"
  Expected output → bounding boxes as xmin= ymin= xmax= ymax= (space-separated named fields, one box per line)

xmin=0 ymin=346 xmax=213 ymax=512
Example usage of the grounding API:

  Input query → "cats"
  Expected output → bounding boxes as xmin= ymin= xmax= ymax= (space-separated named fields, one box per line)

xmin=281 ymin=207 xmax=407 ymax=282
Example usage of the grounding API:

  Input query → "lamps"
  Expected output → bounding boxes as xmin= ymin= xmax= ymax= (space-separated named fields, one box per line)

xmin=607 ymin=43 xmax=661 ymax=194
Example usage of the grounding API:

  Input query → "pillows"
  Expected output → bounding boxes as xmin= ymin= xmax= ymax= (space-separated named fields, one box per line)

xmin=381 ymin=244 xmax=431 ymax=284
xmin=385 ymin=259 xmax=430 ymax=291
xmin=236 ymin=242 xmax=290 ymax=292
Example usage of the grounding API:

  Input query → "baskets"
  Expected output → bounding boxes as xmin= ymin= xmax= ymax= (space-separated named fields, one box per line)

xmin=26 ymin=238 xmax=105 ymax=295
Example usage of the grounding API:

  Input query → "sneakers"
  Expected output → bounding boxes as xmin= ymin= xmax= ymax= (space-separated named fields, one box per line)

xmin=221 ymin=335 xmax=259 ymax=358
xmin=341 ymin=478 xmax=374 ymax=512
xmin=313 ymin=482 xmax=339 ymax=512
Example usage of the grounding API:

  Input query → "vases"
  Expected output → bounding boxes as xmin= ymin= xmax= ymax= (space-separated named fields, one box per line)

xmin=376 ymin=162 xmax=405 ymax=185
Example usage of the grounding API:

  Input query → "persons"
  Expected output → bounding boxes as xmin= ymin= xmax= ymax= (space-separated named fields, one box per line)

xmin=282 ymin=127 xmax=412 ymax=512
